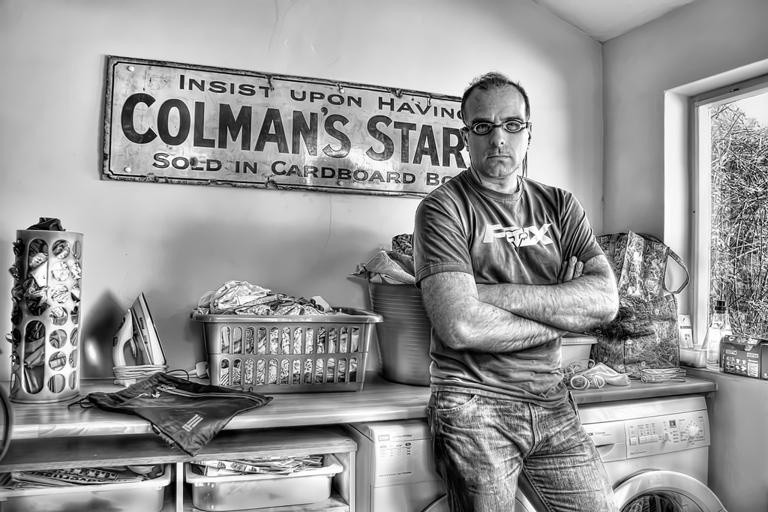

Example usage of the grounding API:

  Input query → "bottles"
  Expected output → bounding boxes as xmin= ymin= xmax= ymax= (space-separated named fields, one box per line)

xmin=706 ymin=300 xmax=733 ymax=372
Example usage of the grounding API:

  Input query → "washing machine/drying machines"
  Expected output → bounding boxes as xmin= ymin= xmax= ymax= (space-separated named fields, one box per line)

xmin=342 ymin=422 xmax=538 ymax=512
xmin=575 ymin=396 xmax=729 ymax=512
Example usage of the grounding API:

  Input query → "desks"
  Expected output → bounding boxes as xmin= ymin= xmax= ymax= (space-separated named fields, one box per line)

xmin=0 ymin=365 xmax=716 ymax=441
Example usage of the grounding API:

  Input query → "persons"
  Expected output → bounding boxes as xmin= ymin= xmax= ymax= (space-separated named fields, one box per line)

xmin=414 ymin=79 xmax=619 ymax=512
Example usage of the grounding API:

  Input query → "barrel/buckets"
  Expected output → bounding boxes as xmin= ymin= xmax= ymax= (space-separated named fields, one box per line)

xmin=346 ymin=262 xmax=434 ymax=386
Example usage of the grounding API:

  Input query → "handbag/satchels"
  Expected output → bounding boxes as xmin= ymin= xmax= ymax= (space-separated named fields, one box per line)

xmin=594 ymin=232 xmax=691 ymax=309
xmin=65 ymin=367 xmax=274 ymax=456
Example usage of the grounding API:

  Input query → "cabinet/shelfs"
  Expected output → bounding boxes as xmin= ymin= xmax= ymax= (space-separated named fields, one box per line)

xmin=0 ymin=431 xmax=358 ymax=512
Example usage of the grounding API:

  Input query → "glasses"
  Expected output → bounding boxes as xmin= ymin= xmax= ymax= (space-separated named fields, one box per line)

xmin=465 ymin=120 xmax=529 ymax=136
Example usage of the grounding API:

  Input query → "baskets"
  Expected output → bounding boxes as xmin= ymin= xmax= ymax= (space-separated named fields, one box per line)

xmin=191 ymin=301 xmax=384 ymax=396
